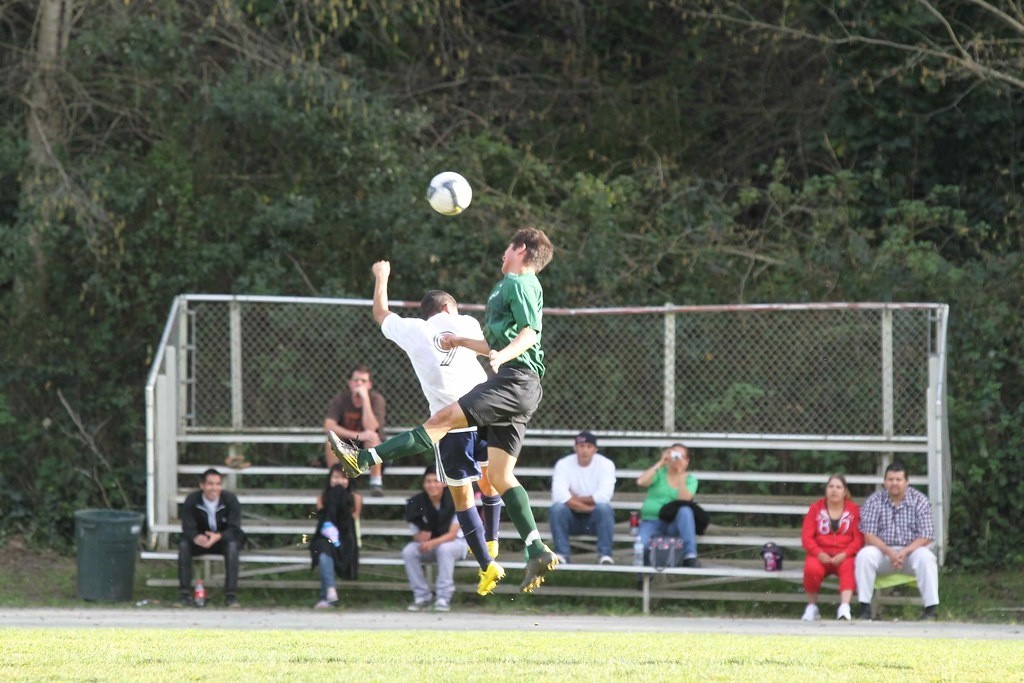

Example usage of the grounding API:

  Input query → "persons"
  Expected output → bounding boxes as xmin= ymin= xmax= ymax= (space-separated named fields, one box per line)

xmin=637 ymin=444 xmax=698 ymax=590
xmin=316 ymin=464 xmax=361 ymax=610
xmin=329 ymin=229 xmax=559 ymax=592
xmin=802 ymin=475 xmax=865 ymax=620
xmin=324 ymin=366 xmax=388 ymax=496
xmin=373 ymin=260 xmax=506 ymax=595
xmin=403 ymin=467 xmax=467 ymax=611
xmin=855 ymin=464 xmax=939 ymax=620
xmin=174 ymin=468 xmax=245 ymax=606
xmin=549 ymin=433 xmax=615 ymax=564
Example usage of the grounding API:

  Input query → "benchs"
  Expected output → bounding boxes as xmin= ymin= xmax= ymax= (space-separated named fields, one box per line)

xmin=136 ymin=433 xmax=931 ymax=607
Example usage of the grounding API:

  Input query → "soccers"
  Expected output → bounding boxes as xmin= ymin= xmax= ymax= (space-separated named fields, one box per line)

xmin=426 ymin=172 xmax=473 ymax=216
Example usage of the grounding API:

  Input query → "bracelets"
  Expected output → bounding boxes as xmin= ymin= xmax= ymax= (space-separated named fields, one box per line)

xmin=654 ymin=464 xmax=659 ymax=472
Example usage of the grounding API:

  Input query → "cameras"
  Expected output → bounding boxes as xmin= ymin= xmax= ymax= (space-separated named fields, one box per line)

xmin=670 ymin=452 xmax=682 ymax=460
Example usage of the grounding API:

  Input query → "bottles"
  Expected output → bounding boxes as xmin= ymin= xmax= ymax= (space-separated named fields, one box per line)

xmin=629 ymin=511 xmax=639 ymax=534
xmin=195 ymin=580 xmax=205 ymax=606
xmin=633 ymin=536 xmax=645 ymax=566
xmin=764 ymin=543 xmax=777 ymax=571
xmin=323 ymin=522 xmax=340 ymax=547
xmin=650 ymin=537 xmax=681 ymax=566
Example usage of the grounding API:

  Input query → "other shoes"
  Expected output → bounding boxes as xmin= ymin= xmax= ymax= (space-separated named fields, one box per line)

xmin=316 ymin=593 xmax=342 ymax=611
xmin=922 ymin=605 xmax=936 ymax=620
xmin=175 ymin=595 xmax=190 ymax=607
xmin=223 ymin=596 xmax=239 ymax=608
xmin=801 ymin=608 xmax=818 ymax=622
xmin=684 ymin=557 xmax=701 ymax=565
xmin=408 ymin=599 xmax=433 ymax=612
xmin=599 ymin=553 xmax=614 ymax=565
xmin=857 ymin=605 xmax=871 ymax=620
xmin=434 ymin=598 xmax=450 ymax=612
xmin=837 ymin=603 xmax=851 ymax=621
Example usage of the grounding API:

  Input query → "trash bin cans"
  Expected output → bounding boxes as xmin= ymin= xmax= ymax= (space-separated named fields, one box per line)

xmin=76 ymin=510 xmax=145 ymax=603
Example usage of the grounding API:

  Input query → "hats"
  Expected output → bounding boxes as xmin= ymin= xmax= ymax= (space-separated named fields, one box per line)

xmin=575 ymin=431 xmax=594 ymax=445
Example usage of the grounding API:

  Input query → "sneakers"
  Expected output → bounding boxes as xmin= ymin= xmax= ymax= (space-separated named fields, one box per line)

xmin=469 ymin=540 xmax=499 ymax=559
xmin=519 ymin=545 xmax=560 ymax=595
xmin=328 ymin=429 xmax=369 ymax=479
xmin=476 ymin=559 xmax=504 ymax=597
